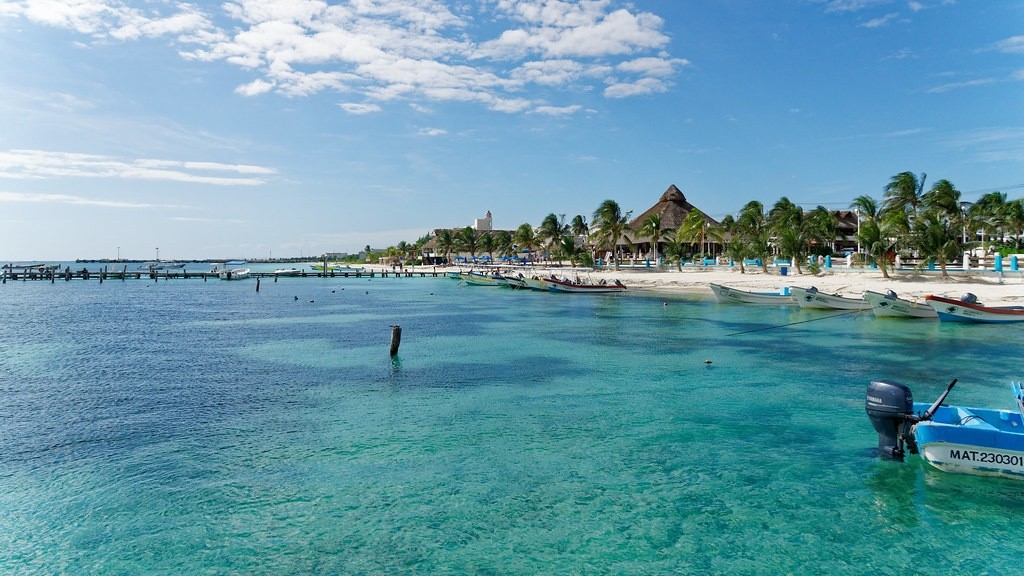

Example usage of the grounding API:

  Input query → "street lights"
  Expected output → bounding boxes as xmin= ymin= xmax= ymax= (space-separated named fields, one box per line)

xmin=652 ymin=222 xmax=656 ymax=261
xmin=854 ymin=208 xmax=861 ymax=253
xmin=706 ymin=223 xmax=711 ymax=258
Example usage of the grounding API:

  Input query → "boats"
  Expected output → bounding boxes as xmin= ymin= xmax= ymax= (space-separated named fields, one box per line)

xmin=273 ymin=269 xmax=301 ymax=274
xmin=446 ymin=272 xmax=461 ymax=279
xmin=219 ymin=267 xmax=250 ymax=280
xmin=788 ymin=285 xmax=873 ymax=310
xmin=491 ymin=269 xmax=627 ymax=294
xmin=310 ymin=265 xmax=348 ymax=270
xmin=2 ymin=262 xmax=61 ymax=271
xmin=708 ymin=283 xmax=798 ymax=305
xmin=861 ymin=289 xmax=938 ymax=318
xmin=866 ymin=378 xmax=1024 ymax=481
xmin=458 ymin=271 xmax=508 ymax=287
xmin=136 ymin=263 xmax=186 ymax=270
xmin=924 ymin=293 xmax=1024 ymax=324
